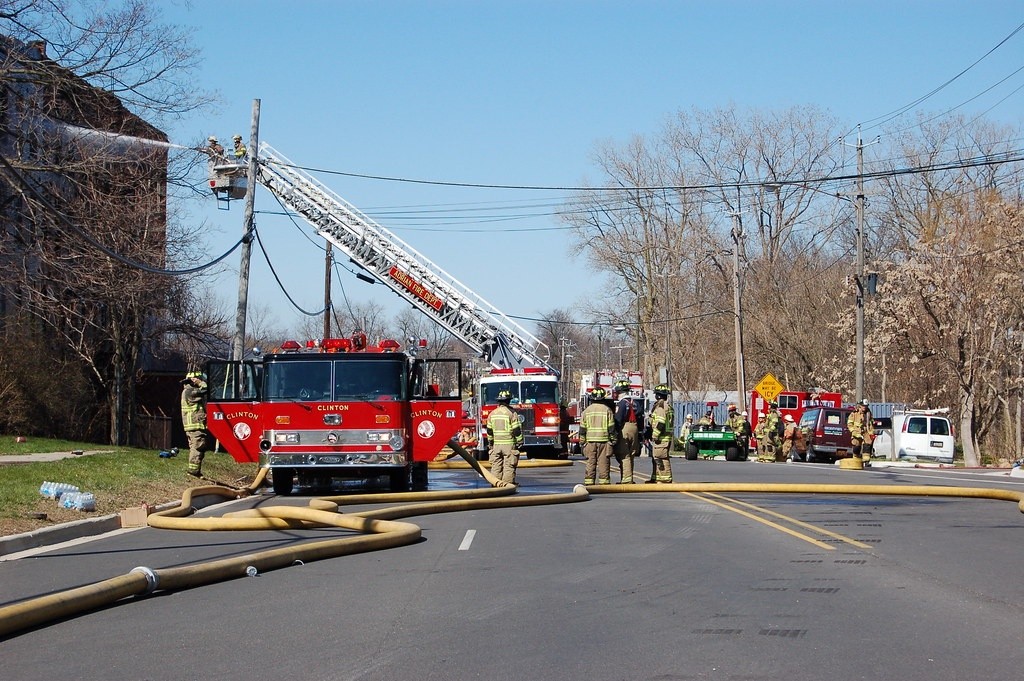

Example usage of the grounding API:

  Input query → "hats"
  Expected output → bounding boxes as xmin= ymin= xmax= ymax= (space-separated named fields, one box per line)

xmin=653 ymin=385 xmax=670 ymax=395
xmin=859 ymin=398 xmax=869 ymax=406
xmin=615 ymin=380 xmax=629 ymax=392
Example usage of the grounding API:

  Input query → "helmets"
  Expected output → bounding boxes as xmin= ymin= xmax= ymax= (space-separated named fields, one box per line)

xmin=495 ymin=391 xmax=514 ymax=400
xmin=729 ymin=404 xmax=739 ymax=411
xmin=742 ymin=411 xmax=748 ymax=416
xmin=758 ymin=412 xmax=765 ymax=418
xmin=180 ymin=371 xmax=201 ymax=384
xmin=784 ymin=414 xmax=794 ymax=421
xmin=686 ymin=414 xmax=692 ymax=419
xmin=591 ymin=387 xmax=605 ymax=400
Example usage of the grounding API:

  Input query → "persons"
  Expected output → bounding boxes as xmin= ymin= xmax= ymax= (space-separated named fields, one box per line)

xmin=723 ymin=403 xmax=797 ymax=464
xmin=614 ymin=380 xmax=640 ymax=485
xmin=198 ymin=135 xmax=247 ymax=178
xmin=180 ymin=371 xmax=208 ymax=480
xmin=487 ymin=389 xmax=521 ymax=487
xmin=580 ymin=389 xmax=617 ymax=486
xmin=457 ymin=410 xmax=470 ymax=442
xmin=847 ymin=398 xmax=875 ymax=467
xmin=645 ymin=385 xmax=674 ymax=483
xmin=558 ymin=405 xmax=570 ymax=460
xmin=679 ymin=411 xmax=716 ymax=448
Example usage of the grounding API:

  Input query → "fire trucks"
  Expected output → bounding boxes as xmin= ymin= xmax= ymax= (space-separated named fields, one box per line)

xmin=566 ymin=367 xmax=645 ymax=457
xmin=207 ymin=328 xmax=464 ymax=495
xmin=208 ymin=141 xmax=567 ymax=459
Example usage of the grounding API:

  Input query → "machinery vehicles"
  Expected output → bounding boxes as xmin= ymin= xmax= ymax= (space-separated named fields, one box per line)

xmin=685 ymin=425 xmax=738 ymax=462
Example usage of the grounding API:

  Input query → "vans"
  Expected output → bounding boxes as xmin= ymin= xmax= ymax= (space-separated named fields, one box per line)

xmin=870 ymin=413 xmax=955 ymax=464
xmin=792 ymin=407 xmax=854 ymax=463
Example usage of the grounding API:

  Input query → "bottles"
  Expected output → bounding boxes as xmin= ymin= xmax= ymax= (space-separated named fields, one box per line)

xmin=41 ymin=481 xmax=95 ymax=511
xmin=246 ymin=566 xmax=257 ymax=576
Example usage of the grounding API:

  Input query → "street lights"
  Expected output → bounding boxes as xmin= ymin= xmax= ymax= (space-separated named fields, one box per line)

xmin=614 ymin=326 xmax=639 ymax=370
xmin=762 ymin=183 xmax=864 ymax=405
xmin=657 ymin=273 xmax=676 ymax=452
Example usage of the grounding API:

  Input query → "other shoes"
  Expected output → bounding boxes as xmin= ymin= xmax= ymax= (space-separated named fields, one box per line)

xmin=187 ymin=470 xmax=203 ymax=478
xmin=865 ymin=462 xmax=871 ymax=467
xmin=645 ymin=479 xmax=657 ymax=483
xmin=746 ymin=457 xmax=751 ymax=461
